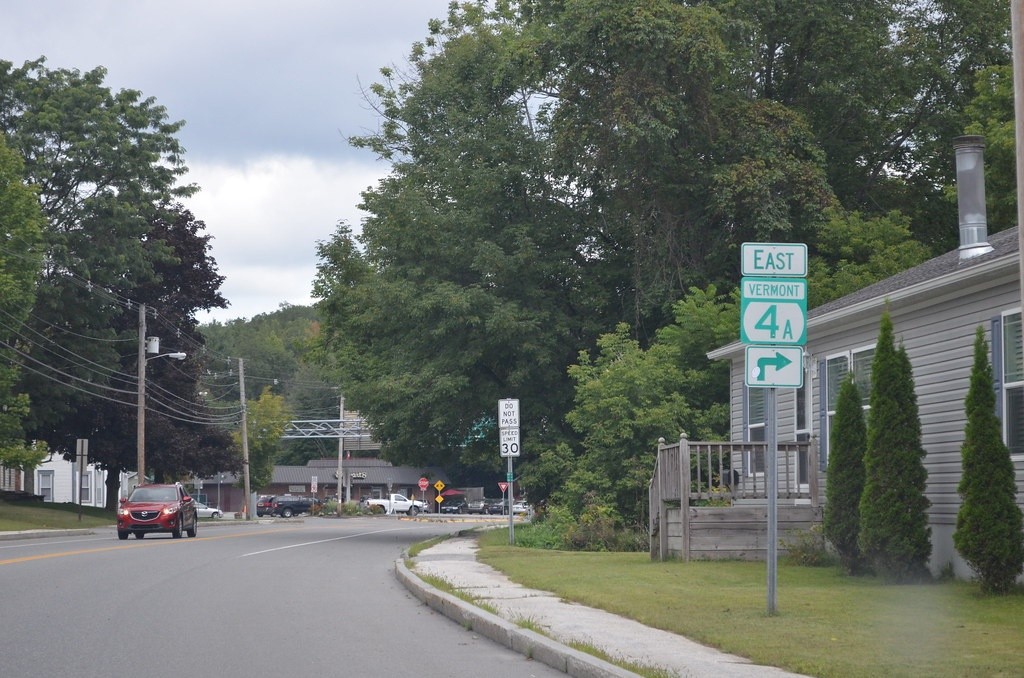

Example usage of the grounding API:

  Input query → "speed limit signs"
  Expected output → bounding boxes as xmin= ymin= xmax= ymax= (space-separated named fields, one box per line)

xmin=500 ymin=428 xmax=520 ymax=458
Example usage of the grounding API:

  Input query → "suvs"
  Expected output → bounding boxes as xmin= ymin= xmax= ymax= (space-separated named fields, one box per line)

xmin=116 ymin=482 xmax=199 ymax=539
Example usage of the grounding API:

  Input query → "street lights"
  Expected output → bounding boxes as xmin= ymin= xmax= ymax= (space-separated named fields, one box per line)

xmin=137 ymin=351 xmax=187 ymax=482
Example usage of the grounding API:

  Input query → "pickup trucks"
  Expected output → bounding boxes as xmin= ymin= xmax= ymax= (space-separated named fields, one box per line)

xmin=364 ymin=493 xmax=429 ymax=516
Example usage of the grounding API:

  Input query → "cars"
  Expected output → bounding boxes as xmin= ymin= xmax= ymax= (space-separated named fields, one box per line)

xmin=488 ymin=498 xmax=533 ymax=516
xmin=195 ymin=500 xmax=223 ymax=519
xmin=255 ymin=495 xmax=324 ymax=518
xmin=468 ymin=498 xmax=491 ymax=514
xmin=441 ymin=499 xmax=469 ymax=514
